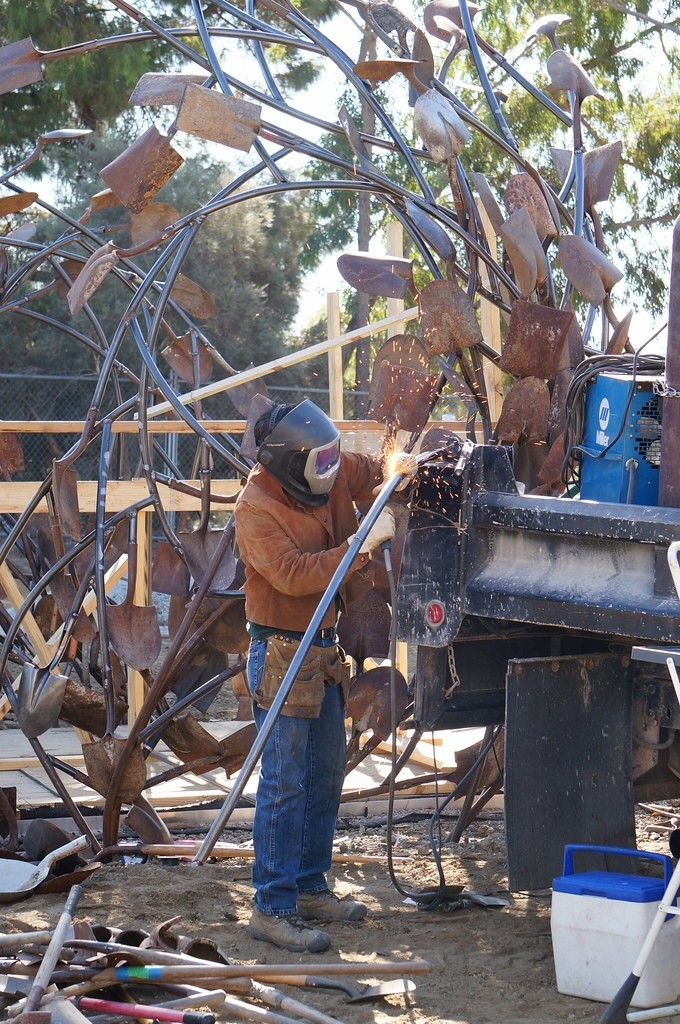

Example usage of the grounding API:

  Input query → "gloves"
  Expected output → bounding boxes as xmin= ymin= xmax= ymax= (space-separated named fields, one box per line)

xmin=347 ymin=506 xmax=396 ymax=560
xmin=372 ymin=451 xmax=418 ymax=496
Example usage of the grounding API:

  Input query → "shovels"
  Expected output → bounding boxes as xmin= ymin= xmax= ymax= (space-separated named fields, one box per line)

xmin=0 ymin=883 xmax=82 ymax=1024
xmin=1 ymin=0 xmax=649 ymax=893
xmin=249 ymin=973 xmax=418 ymax=1005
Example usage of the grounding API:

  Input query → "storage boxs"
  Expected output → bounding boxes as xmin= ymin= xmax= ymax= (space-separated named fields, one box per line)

xmin=550 ymin=843 xmax=680 ymax=1009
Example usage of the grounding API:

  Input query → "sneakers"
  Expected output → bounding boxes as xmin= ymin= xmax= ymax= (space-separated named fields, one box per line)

xmin=297 ymin=889 xmax=367 ymax=921
xmin=249 ymin=905 xmax=331 ymax=953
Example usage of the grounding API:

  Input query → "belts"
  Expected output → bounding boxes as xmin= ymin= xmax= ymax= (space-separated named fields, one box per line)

xmin=277 ymin=628 xmax=335 ymax=641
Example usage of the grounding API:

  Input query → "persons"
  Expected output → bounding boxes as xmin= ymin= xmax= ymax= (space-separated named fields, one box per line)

xmin=234 ymin=401 xmax=418 ymax=951
